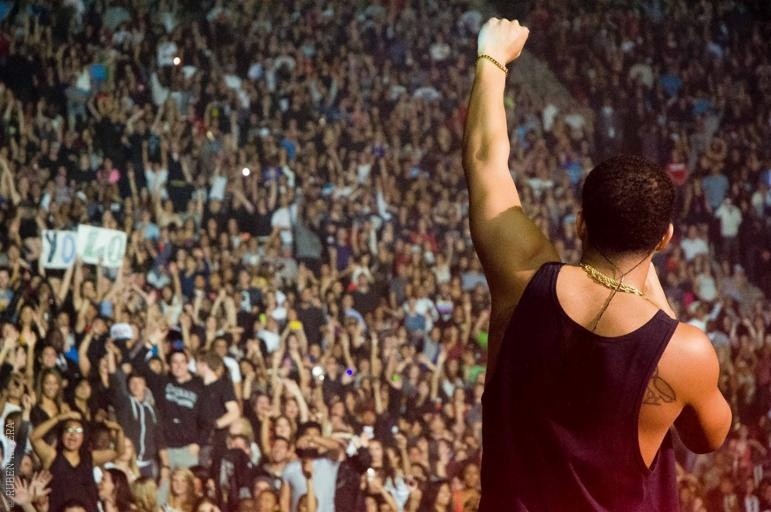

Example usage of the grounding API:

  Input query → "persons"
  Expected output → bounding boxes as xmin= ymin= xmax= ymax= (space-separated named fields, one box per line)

xmin=489 ymin=1 xmax=771 ymax=264
xmin=653 ymin=257 xmax=771 ymax=512
xmin=467 ymin=18 xmax=734 ymax=512
xmin=2 ymin=1 xmax=490 ymax=512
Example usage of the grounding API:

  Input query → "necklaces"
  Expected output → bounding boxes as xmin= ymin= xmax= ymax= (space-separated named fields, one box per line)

xmin=578 ymin=260 xmax=645 ymax=295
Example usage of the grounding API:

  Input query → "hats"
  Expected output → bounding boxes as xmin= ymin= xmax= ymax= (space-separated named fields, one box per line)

xmin=111 ymin=323 xmax=134 ymax=342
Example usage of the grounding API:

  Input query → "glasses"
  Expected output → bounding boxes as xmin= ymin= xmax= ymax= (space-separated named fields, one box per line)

xmin=65 ymin=427 xmax=85 ymax=434
